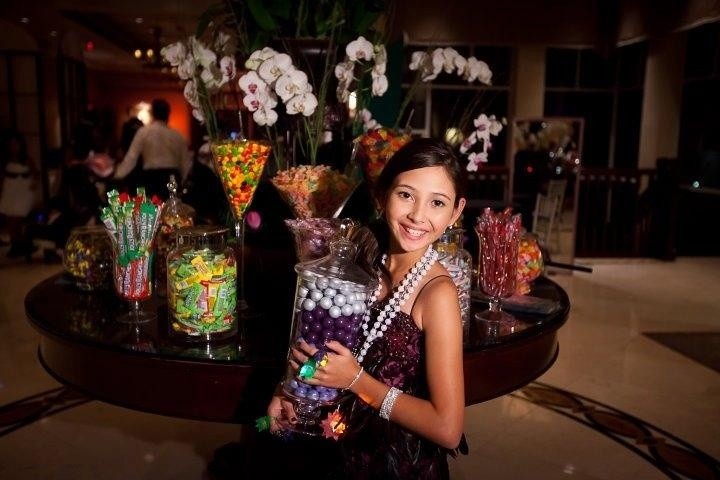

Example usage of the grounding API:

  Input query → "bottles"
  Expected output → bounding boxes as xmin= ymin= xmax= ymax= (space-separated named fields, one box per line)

xmin=63 ymin=228 xmax=112 ymax=292
xmin=165 ymin=227 xmax=238 ymax=343
xmin=436 ymin=228 xmax=473 ymax=330
xmin=157 ymin=173 xmax=198 ymax=290
xmin=516 ymin=226 xmax=542 ymax=296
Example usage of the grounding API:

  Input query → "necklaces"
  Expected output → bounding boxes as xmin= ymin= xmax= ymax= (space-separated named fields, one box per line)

xmin=351 ymin=244 xmax=439 ymax=363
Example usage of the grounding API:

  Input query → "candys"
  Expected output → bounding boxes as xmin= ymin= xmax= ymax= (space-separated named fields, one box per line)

xmin=360 ymin=126 xmax=414 ymax=189
xmin=63 ymin=229 xmax=112 ymax=290
xmin=211 ymin=141 xmax=270 ymax=218
xmin=100 ymin=187 xmax=194 ymax=301
xmin=166 ymin=247 xmax=238 ymax=335
xmin=273 ymin=164 xmax=360 ymax=261
xmin=282 ymin=266 xmax=371 ymax=404
xmin=435 ymin=214 xmax=543 ymax=330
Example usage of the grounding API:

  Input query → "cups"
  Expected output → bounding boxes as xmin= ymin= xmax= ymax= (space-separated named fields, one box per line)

xmin=286 ymin=218 xmax=361 ymax=263
xmin=270 ymin=167 xmax=366 ymax=219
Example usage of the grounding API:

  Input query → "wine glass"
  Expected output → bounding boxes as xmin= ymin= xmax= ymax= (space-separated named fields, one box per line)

xmin=475 ymin=215 xmax=521 ymax=326
xmin=277 ymin=239 xmax=380 ymax=437
xmin=103 ymin=227 xmax=158 ymax=326
xmin=208 ymin=137 xmax=274 ymax=310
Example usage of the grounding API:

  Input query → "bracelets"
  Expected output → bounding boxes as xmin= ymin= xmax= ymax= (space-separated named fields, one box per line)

xmin=378 ymin=386 xmax=404 ymax=421
xmin=340 ymin=365 xmax=365 ymax=393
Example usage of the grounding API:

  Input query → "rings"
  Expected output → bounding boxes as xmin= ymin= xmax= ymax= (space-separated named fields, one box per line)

xmin=312 ymin=348 xmax=329 ymax=367
xmin=255 ymin=416 xmax=277 ymax=431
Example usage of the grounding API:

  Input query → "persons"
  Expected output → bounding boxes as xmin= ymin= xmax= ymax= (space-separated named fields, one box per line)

xmin=112 ymin=117 xmax=145 ymax=198
xmin=209 ymin=135 xmax=471 ymax=480
xmin=111 ymin=96 xmax=189 ymax=212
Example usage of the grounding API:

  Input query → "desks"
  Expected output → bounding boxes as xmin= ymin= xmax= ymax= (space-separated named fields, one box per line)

xmin=23 ymin=270 xmax=570 ymax=440
